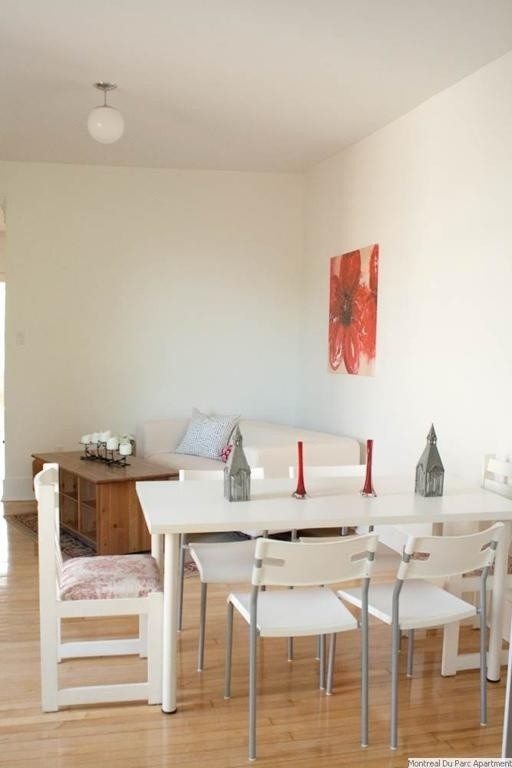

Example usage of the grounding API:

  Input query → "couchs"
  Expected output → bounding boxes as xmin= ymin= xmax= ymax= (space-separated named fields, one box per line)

xmin=133 ymin=415 xmax=362 ymax=475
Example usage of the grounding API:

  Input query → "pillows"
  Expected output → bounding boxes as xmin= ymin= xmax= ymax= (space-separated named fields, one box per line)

xmin=176 ymin=403 xmax=243 ymax=463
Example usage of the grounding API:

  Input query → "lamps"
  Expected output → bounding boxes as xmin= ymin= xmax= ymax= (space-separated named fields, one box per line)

xmin=84 ymin=80 xmax=125 ymax=146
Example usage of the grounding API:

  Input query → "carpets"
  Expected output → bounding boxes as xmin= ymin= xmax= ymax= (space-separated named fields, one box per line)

xmin=3 ymin=513 xmax=96 ymax=558
